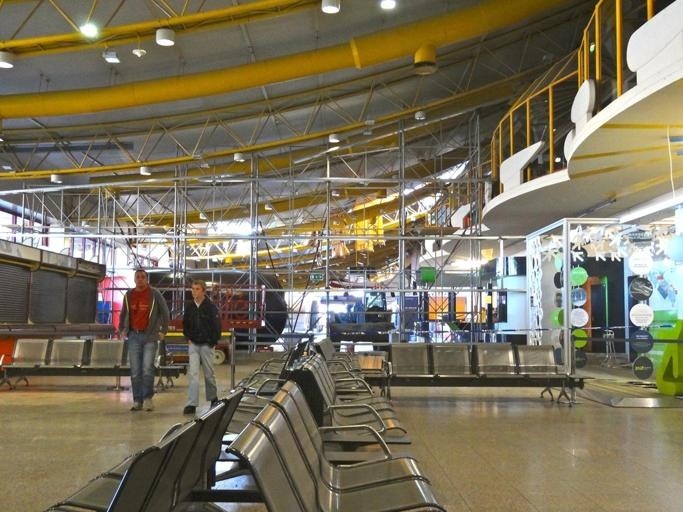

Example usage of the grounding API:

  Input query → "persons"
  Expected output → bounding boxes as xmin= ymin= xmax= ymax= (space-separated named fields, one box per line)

xmin=181 ymin=279 xmax=221 ymax=416
xmin=118 ymin=269 xmax=171 ymax=412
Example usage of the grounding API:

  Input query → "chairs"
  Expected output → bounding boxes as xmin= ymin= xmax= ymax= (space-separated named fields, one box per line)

xmin=1 ymin=338 xmax=50 ymax=390
xmin=268 ymin=391 xmax=445 ymax=512
xmin=173 ymin=403 xmax=226 ymax=512
xmin=99 ymin=421 xmax=202 ymax=512
xmin=430 ymin=343 xmax=477 ymax=385
xmin=251 ymin=403 xmax=318 ymax=512
xmin=515 ymin=345 xmax=570 ymax=407
xmin=118 ymin=340 xmax=185 ymax=393
xmin=82 ymin=339 xmax=127 ymax=377
xmin=228 ymin=340 xmax=413 ymax=438
xmin=472 ymin=343 xmax=524 ymax=387
xmin=41 ymin=340 xmax=89 ymax=376
xmin=269 ymin=380 xmax=431 ymax=490
xmin=225 ymin=421 xmax=304 ymax=512
xmin=217 ymin=389 xmax=244 ymax=440
xmin=391 ymin=344 xmax=435 ymax=384
xmin=47 ymin=437 xmax=175 ymax=512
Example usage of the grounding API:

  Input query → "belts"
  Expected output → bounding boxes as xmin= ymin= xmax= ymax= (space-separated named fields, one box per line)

xmin=130 ymin=328 xmax=146 ymax=334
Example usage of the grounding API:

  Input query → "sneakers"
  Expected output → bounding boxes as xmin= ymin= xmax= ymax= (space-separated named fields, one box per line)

xmin=183 ymin=406 xmax=196 ymax=415
xmin=211 ymin=397 xmax=218 ymax=407
xmin=143 ymin=398 xmax=155 ymax=412
xmin=129 ymin=402 xmax=144 ymax=411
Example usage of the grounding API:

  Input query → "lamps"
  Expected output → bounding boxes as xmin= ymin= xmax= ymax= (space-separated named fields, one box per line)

xmin=265 ymin=202 xmax=274 ymax=212
xmin=322 ymin=0 xmax=343 ymax=15
xmin=102 ymin=43 xmax=127 ymax=65
xmin=199 ymin=211 xmax=209 ymax=222
xmin=2 ymin=50 xmax=15 ymax=71
xmin=327 ymin=134 xmax=341 ymax=144
xmin=154 ymin=25 xmax=177 ymax=50
xmin=380 ymin=1 xmax=398 ymax=12
xmin=414 ymin=111 xmax=427 ymax=123
xmin=233 ymin=153 xmax=246 ymax=164
xmin=80 ymin=17 xmax=101 ymax=45
xmin=50 ymin=174 xmax=63 ymax=185
xmin=139 ymin=166 xmax=153 ymax=177
xmin=331 ymin=187 xmax=341 ymax=198
